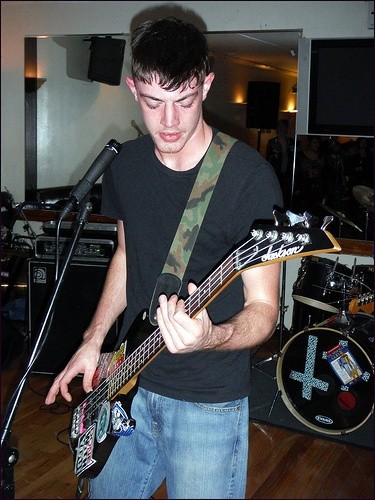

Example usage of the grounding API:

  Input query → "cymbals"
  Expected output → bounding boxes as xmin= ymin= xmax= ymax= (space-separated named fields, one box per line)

xmin=352 ymin=185 xmax=375 ymax=214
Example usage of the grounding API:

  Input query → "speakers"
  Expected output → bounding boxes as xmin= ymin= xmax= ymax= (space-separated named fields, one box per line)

xmin=88 ymin=35 xmax=125 ymax=86
xmin=25 ymin=259 xmax=120 ymax=377
xmin=247 ymin=80 xmax=281 ymax=129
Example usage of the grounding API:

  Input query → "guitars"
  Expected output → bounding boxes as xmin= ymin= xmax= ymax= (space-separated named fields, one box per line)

xmin=67 ymin=209 xmax=343 ymax=478
xmin=348 ymin=292 xmax=375 ymax=315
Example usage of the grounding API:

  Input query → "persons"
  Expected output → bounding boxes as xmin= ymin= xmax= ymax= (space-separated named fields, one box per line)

xmin=266 ymin=120 xmax=375 ymax=214
xmin=44 ymin=16 xmax=284 ymax=499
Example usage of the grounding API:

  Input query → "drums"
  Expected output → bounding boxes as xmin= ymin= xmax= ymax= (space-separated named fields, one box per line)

xmin=276 ymin=311 xmax=375 ymax=435
xmin=352 ymin=264 xmax=375 ymax=292
xmin=292 ymin=255 xmax=360 ymax=314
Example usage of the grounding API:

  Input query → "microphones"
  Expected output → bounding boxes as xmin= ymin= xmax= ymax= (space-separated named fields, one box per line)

xmin=59 ymin=140 xmax=121 ymax=219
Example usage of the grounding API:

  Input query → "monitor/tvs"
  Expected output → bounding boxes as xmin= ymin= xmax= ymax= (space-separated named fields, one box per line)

xmin=307 ymin=38 xmax=375 ymax=136
xmin=293 ymin=134 xmax=375 ymax=241
